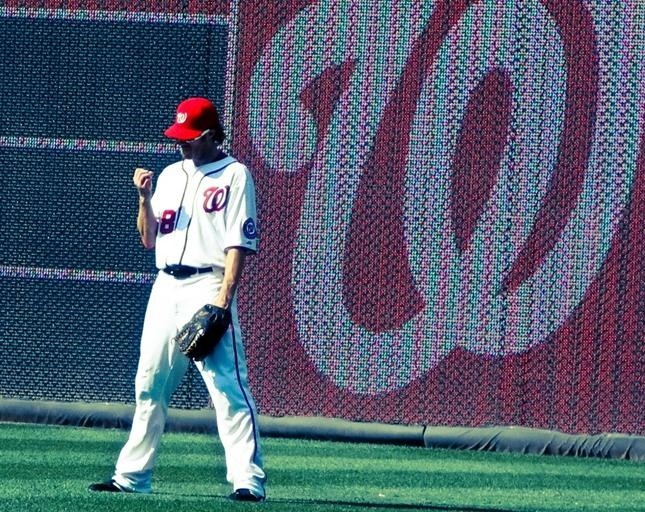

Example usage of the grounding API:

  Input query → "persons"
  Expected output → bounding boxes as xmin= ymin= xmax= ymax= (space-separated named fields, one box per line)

xmin=89 ymin=97 xmax=266 ymax=500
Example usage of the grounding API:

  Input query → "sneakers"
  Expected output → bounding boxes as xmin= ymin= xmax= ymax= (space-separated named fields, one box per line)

xmin=229 ymin=489 xmax=266 ymax=502
xmin=87 ymin=481 xmax=152 ymax=492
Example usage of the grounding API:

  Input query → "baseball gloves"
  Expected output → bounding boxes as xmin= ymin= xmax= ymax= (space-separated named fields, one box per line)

xmin=175 ymin=303 xmax=231 ymax=361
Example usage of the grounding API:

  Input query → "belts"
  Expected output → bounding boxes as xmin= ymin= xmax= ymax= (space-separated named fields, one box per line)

xmin=162 ymin=265 xmax=211 ymax=275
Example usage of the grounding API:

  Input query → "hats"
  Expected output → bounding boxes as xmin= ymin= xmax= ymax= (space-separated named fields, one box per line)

xmin=163 ymin=99 xmax=222 ymax=141
